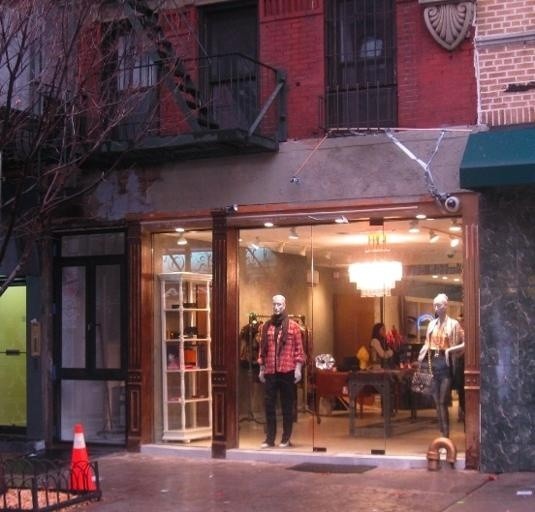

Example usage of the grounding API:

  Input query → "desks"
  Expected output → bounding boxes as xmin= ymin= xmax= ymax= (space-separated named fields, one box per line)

xmin=316 ymin=368 xmax=414 ymax=439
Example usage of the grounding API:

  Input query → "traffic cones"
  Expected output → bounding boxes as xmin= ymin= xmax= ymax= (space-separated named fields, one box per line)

xmin=68 ymin=423 xmax=97 ymax=491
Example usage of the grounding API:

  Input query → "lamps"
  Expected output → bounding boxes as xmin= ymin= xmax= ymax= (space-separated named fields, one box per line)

xmin=409 ymin=219 xmax=461 ymax=247
xmin=289 ymin=227 xmax=299 ymax=240
xmin=176 ymin=232 xmax=187 ymax=245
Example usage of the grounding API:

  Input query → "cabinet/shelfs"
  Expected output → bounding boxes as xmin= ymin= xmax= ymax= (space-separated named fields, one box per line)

xmin=157 ymin=272 xmax=213 ymax=444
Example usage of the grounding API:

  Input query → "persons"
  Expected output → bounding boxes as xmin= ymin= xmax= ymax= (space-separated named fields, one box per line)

xmin=258 ymin=294 xmax=308 ymax=448
xmin=418 ymin=292 xmax=465 ymax=455
xmin=368 ymin=321 xmax=396 ymax=419
